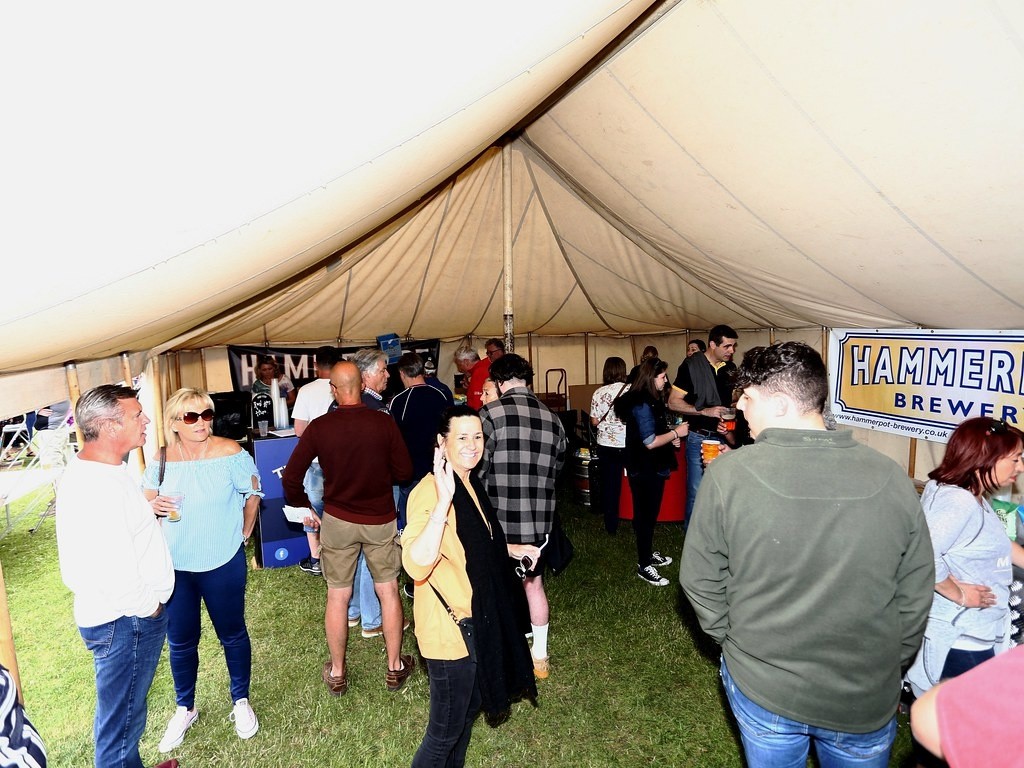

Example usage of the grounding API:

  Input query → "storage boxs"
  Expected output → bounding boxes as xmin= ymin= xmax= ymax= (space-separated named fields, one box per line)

xmin=570 ymin=383 xmax=604 ymax=440
xmin=375 ymin=332 xmax=402 ymax=366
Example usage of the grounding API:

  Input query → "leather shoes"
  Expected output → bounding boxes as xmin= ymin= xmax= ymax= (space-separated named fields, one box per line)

xmin=386 ymin=655 xmax=415 ymax=692
xmin=323 ymin=660 xmax=347 ymax=696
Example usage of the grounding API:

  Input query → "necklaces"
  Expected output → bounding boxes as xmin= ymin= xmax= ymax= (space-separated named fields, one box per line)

xmin=177 ymin=438 xmax=209 ymax=462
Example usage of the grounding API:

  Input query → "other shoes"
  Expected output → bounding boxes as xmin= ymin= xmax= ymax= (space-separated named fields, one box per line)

xmin=531 ymin=651 xmax=550 ymax=679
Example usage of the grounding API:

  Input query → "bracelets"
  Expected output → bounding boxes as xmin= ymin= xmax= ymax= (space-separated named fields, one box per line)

xmin=670 ymin=429 xmax=678 ymax=439
xmin=430 ymin=514 xmax=449 ymax=525
xmin=725 ymin=432 xmax=729 ymax=435
xmin=957 ymin=587 xmax=965 ymax=610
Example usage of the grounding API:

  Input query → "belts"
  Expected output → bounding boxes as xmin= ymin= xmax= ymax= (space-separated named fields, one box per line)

xmin=689 ymin=427 xmax=719 ymax=437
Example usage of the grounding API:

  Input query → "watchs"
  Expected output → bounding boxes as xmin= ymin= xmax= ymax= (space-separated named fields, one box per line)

xmin=243 ymin=535 xmax=249 ymax=543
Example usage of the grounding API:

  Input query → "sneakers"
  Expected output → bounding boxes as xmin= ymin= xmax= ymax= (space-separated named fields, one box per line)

xmin=299 ymin=559 xmax=321 ymax=575
xmin=158 ymin=704 xmax=198 ymax=753
xmin=638 ymin=566 xmax=669 ymax=586
xmin=233 ymin=698 xmax=259 ymax=739
xmin=348 ymin=617 xmax=360 ymax=627
xmin=362 ymin=619 xmax=410 ymax=638
xmin=154 ymin=759 xmax=179 ymax=768
xmin=638 ymin=555 xmax=672 ymax=567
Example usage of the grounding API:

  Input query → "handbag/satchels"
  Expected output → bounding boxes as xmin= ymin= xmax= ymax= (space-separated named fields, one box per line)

xmin=458 ymin=617 xmax=477 ymax=663
xmin=545 ymin=532 xmax=573 ymax=575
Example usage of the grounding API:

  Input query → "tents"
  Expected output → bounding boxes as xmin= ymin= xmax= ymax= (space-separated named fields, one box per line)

xmin=0 ymin=0 xmax=1024 ymax=474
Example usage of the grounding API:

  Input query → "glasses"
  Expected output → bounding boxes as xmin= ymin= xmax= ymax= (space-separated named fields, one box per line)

xmin=515 ymin=534 xmax=549 ymax=577
xmin=486 ymin=349 xmax=503 ymax=355
xmin=985 ymin=418 xmax=1010 ymax=437
xmin=171 ymin=409 xmax=213 ymax=424
xmin=655 ymin=356 xmax=661 ymax=366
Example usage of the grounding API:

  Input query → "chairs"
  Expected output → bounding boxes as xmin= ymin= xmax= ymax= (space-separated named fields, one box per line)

xmin=0 ymin=407 xmax=77 ymax=541
xmin=210 ymin=391 xmax=255 ymax=454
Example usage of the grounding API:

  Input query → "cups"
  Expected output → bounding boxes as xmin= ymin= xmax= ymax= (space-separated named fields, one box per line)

xmin=258 ymin=420 xmax=268 ymax=436
xmin=702 ymin=439 xmax=721 ymax=463
xmin=271 ymin=378 xmax=289 ymax=431
xmin=165 ymin=490 xmax=185 ymax=523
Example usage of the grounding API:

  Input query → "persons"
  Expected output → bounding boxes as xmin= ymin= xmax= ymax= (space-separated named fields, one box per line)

xmin=679 ymin=341 xmax=936 ymax=768
xmin=400 ymin=406 xmax=541 ymax=768
xmin=0 ymin=562 xmax=48 ymax=768
xmin=0 ymin=405 xmax=75 ymax=462
xmin=254 ymin=340 xmax=568 ymax=694
xmin=904 ymin=417 xmax=1024 ymax=768
xmin=142 ymin=389 xmax=264 ymax=754
xmin=590 ymin=324 xmax=755 ymax=587
xmin=56 ymin=384 xmax=178 ymax=768
